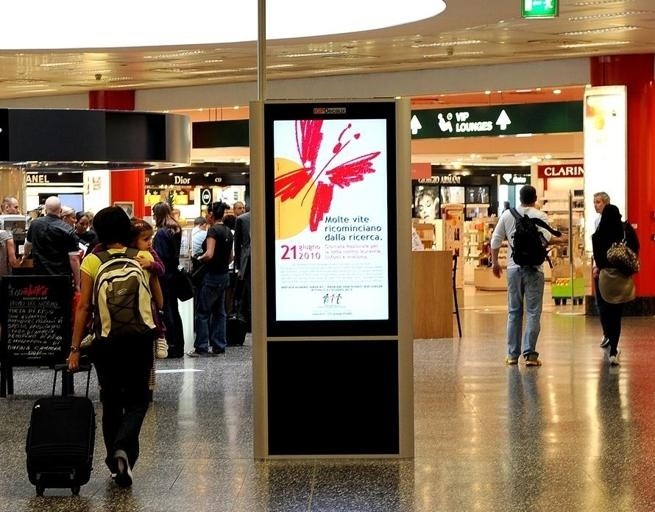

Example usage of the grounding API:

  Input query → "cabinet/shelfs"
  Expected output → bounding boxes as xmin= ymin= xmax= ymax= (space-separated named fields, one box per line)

xmin=411 ymin=188 xmax=585 ymax=339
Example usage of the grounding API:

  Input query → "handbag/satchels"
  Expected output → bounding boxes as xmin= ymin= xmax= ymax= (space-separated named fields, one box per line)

xmin=606 ymin=239 xmax=640 ymax=272
xmin=176 ymin=267 xmax=195 ymax=301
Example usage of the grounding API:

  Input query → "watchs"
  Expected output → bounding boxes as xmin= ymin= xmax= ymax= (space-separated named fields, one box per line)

xmin=68 ymin=345 xmax=81 ymax=354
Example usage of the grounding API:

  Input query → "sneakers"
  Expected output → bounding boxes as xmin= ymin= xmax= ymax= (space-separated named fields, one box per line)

xmin=526 ymin=357 xmax=542 ymax=366
xmin=608 ymin=348 xmax=620 ymax=365
xmin=505 ymin=357 xmax=517 ymax=364
xmin=80 ymin=332 xmax=95 ymax=348
xmin=156 ymin=338 xmax=169 ymax=358
xmin=600 ymin=336 xmax=610 ymax=348
xmin=110 ymin=449 xmax=132 ymax=486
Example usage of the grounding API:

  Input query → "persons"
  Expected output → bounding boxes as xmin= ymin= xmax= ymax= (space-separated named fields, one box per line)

xmin=489 ymin=185 xmax=555 ymax=367
xmin=79 ymin=213 xmax=172 ymax=359
xmin=150 ymin=201 xmax=186 ymax=359
xmin=592 ymin=192 xmax=611 ymax=349
xmin=0 ymin=196 xmax=99 ymax=301
xmin=66 ymin=204 xmax=165 ymax=489
xmin=181 ymin=196 xmax=251 ymax=359
xmin=415 ymin=186 xmax=440 ymax=221
xmin=590 ymin=201 xmax=641 ymax=367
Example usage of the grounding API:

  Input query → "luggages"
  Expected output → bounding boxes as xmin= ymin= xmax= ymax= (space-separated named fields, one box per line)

xmin=226 ymin=290 xmax=247 ymax=346
xmin=26 ymin=364 xmax=97 ymax=495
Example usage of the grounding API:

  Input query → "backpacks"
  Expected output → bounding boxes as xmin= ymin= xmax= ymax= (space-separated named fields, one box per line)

xmin=91 ymin=248 xmax=156 ymax=340
xmin=510 ymin=208 xmax=561 ymax=268
